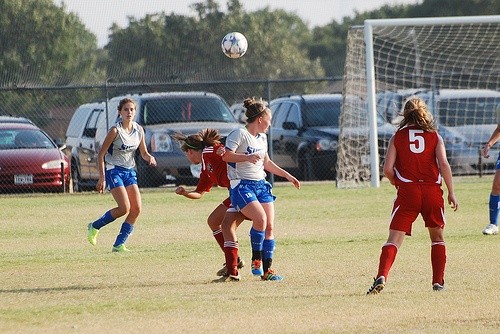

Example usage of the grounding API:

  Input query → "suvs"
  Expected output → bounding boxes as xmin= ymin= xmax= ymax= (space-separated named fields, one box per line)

xmin=224 ymin=89 xmax=500 ymax=182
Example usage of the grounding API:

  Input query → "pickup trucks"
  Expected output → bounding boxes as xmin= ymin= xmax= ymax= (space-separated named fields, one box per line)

xmin=65 ymin=92 xmax=243 ymax=193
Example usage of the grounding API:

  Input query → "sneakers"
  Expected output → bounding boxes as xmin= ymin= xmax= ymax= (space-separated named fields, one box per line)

xmin=111 ymin=244 xmax=130 ymax=252
xmin=433 ymin=280 xmax=447 ymax=291
xmin=251 ymin=256 xmax=262 ymax=275
xmin=261 ymin=268 xmax=283 ymax=280
xmin=211 ymin=272 xmax=239 ymax=283
xmin=366 ymin=276 xmax=385 ymax=295
xmin=216 ymin=256 xmax=244 ymax=276
xmin=482 ymin=224 xmax=499 ymax=235
xmin=87 ymin=223 xmax=100 ymax=245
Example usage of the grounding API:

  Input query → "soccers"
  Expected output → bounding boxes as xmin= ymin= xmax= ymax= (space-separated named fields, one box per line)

xmin=221 ymin=31 xmax=248 ymax=59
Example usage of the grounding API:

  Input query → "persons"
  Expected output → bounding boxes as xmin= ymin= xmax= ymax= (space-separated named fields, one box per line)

xmin=174 ymin=128 xmax=252 ymax=283
xmin=87 ymin=98 xmax=157 ymax=253
xmin=366 ymin=98 xmax=458 ymax=295
xmin=482 ymin=123 xmax=500 ymax=234
xmin=222 ymin=98 xmax=300 ymax=281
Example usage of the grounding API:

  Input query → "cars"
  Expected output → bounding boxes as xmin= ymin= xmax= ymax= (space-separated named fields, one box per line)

xmin=0 ymin=116 xmax=70 ymax=194
